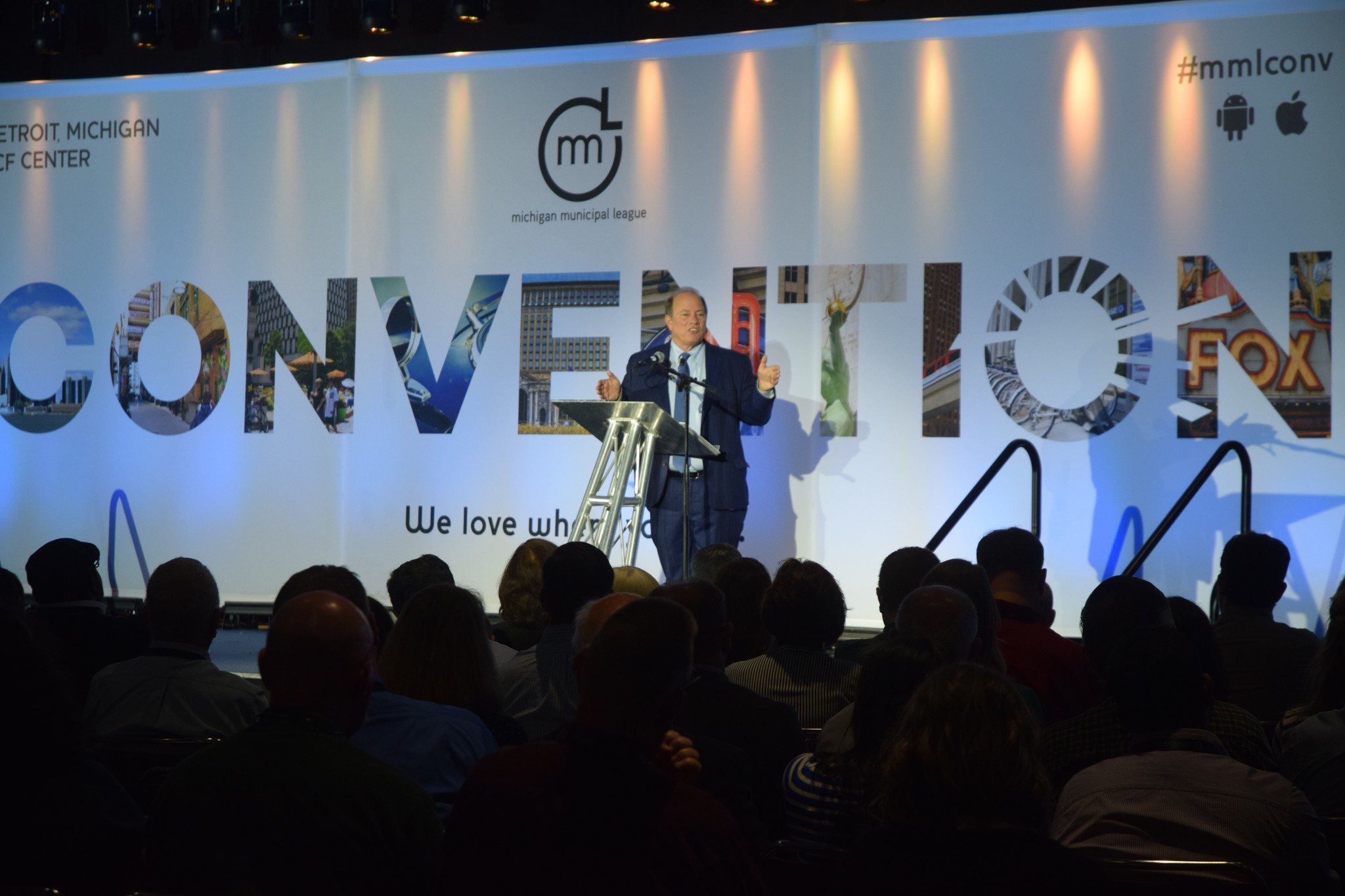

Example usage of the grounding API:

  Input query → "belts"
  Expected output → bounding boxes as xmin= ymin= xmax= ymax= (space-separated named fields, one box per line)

xmin=667 ymin=470 xmax=705 ymax=480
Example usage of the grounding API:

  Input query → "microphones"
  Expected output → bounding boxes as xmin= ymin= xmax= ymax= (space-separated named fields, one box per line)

xmin=636 ymin=350 xmax=667 ymax=369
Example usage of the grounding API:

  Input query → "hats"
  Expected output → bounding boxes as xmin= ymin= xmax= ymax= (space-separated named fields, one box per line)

xmin=1050 ymin=753 xmax=1320 ymax=896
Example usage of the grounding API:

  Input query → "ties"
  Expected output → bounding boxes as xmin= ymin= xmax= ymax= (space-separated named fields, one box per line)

xmin=673 ymin=352 xmax=691 ymax=474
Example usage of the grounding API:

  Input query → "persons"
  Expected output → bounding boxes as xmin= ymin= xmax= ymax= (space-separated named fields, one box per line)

xmin=596 ymin=287 xmax=778 ymax=583
xmin=0 ymin=527 xmax=1344 ymax=896
